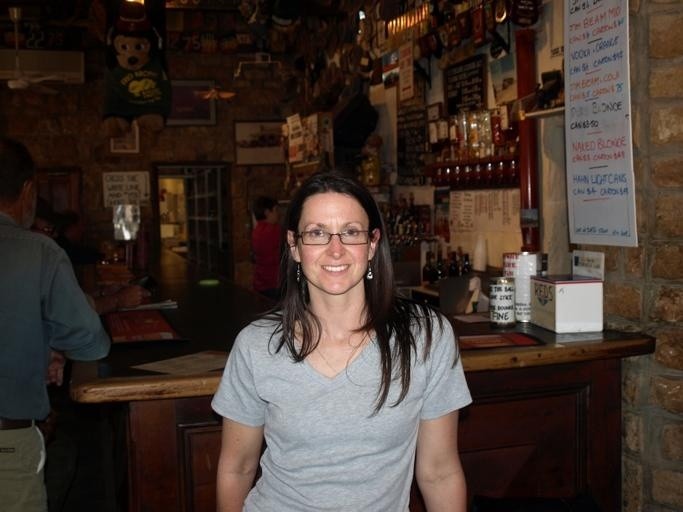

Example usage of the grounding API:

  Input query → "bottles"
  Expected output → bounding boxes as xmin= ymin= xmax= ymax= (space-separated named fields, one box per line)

xmin=422 ymin=245 xmax=473 ymax=291
xmin=434 ymin=159 xmax=518 ymax=187
xmin=385 ymin=210 xmax=426 ymax=244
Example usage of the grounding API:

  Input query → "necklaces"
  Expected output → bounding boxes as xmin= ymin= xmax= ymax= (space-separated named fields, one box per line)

xmin=311 ymin=338 xmax=364 ymax=375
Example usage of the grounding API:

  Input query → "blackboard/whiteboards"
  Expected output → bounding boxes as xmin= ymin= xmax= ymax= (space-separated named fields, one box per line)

xmin=564 ymin=2 xmax=637 ymax=248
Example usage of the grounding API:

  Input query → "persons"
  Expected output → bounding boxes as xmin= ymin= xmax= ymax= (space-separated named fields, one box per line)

xmin=205 ymin=173 xmax=477 ymax=512
xmin=246 ymin=192 xmax=289 ymax=301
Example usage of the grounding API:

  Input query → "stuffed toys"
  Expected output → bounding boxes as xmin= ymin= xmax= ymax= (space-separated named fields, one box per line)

xmin=89 ymin=0 xmax=176 ymax=140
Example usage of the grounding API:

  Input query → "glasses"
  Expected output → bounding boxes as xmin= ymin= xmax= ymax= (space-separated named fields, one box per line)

xmin=296 ymin=228 xmax=372 ymax=247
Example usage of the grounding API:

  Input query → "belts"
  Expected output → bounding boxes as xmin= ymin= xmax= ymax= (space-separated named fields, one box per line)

xmin=0 ymin=417 xmax=38 ymax=430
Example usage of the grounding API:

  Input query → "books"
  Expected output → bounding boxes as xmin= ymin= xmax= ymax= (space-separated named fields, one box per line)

xmin=117 ymin=296 xmax=179 ymax=315
